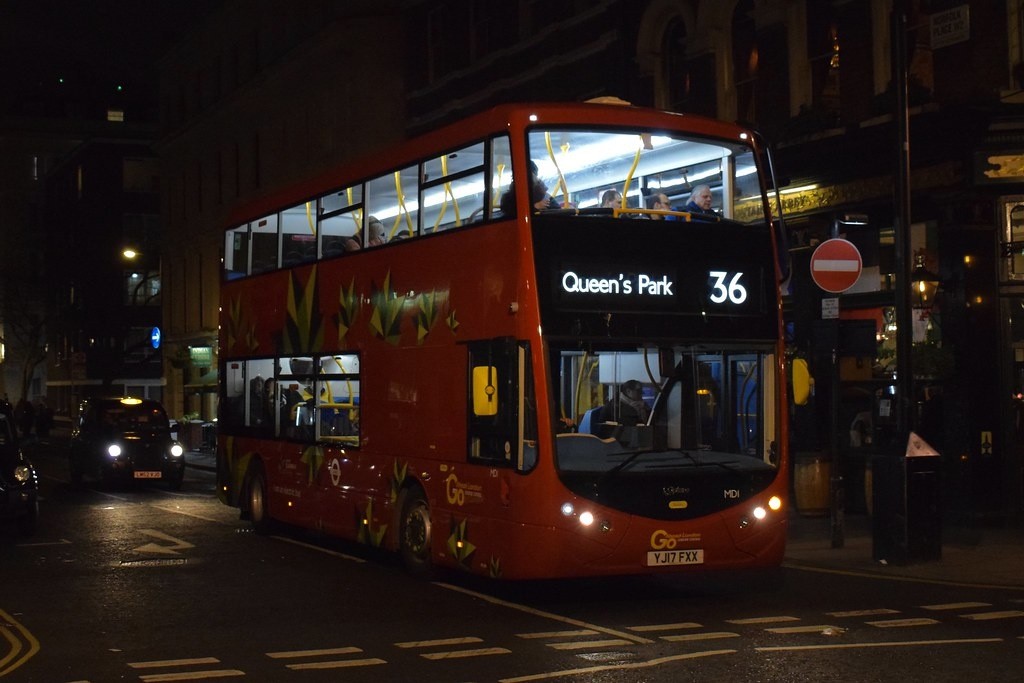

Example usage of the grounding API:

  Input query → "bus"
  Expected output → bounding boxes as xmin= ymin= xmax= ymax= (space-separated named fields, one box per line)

xmin=215 ymin=96 xmax=815 ymax=582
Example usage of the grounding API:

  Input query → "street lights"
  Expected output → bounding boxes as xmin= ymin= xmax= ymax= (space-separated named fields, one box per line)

xmin=123 ymin=250 xmax=147 ymax=304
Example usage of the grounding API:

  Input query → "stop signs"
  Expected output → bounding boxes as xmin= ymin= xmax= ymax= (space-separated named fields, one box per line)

xmin=810 ymin=238 xmax=863 ymax=293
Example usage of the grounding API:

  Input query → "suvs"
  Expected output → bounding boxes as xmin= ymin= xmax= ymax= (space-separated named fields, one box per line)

xmin=67 ymin=394 xmax=184 ymax=491
xmin=0 ymin=397 xmax=42 ymax=537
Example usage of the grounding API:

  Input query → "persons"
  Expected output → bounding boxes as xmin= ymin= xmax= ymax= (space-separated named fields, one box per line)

xmin=597 ymin=380 xmax=651 ymax=440
xmin=593 ymin=183 xmax=723 ymax=222
xmin=98 ymin=413 xmax=118 ymax=431
xmin=239 ymin=376 xmax=360 ymax=444
xmin=345 ymin=216 xmax=411 ymax=252
xmin=850 ymin=384 xmax=945 ymax=451
xmin=500 ymin=160 xmax=561 ymax=216
xmin=0 ymin=397 xmax=26 ymax=431
xmin=524 ymin=378 xmax=575 ymax=440
xmin=559 ymin=201 xmax=580 ymax=216
